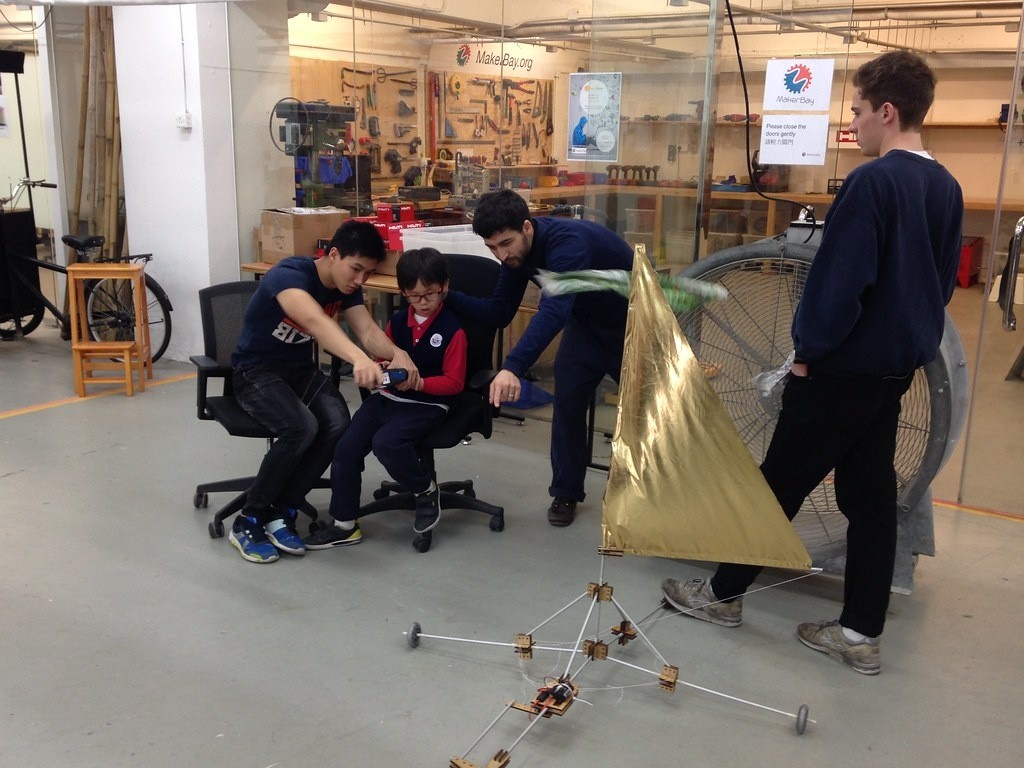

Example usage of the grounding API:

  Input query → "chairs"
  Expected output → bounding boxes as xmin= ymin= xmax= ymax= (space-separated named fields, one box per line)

xmin=189 ymin=280 xmax=332 ymax=539
xmin=357 ymin=254 xmax=503 ymax=552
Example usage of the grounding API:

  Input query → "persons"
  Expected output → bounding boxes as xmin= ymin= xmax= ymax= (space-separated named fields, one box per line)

xmin=228 ymin=221 xmax=420 ymax=563
xmin=660 ymin=50 xmax=964 ymax=675
xmin=304 ymin=247 xmax=466 ymax=549
xmin=573 ymin=116 xmax=598 ymax=148
xmin=472 ymin=188 xmax=634 ymax=527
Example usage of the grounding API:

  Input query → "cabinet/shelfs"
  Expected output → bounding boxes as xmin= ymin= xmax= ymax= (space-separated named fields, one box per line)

xmin=515 ymin=184 xmax=615 ymax=223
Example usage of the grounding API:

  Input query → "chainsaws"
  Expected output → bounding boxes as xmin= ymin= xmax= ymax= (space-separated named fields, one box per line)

xmin=367 ymin=142 xmax=382 ymax=173
xmin=369 ymin=116 xmax=381 ymax=136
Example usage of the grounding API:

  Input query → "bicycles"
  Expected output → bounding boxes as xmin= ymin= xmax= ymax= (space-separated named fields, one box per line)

xmin=0 ymin=179 xmax=174 ymax=368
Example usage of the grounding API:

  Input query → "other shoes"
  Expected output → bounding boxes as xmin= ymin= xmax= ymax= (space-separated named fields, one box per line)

xmin=548 ymin=496 xmax=577 ymax=527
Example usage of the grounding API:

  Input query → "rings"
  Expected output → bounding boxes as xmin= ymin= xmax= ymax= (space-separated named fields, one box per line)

xmin=509 ymin=394 xmax=514 ymax=397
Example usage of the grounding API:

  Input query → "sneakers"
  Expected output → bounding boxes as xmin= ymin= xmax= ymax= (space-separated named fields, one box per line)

xmin=662 ymin=576 xmax=744 ymax=627
xmin=302 ymin=518 xmax=363 ymax=550
xmin=413 ymin=484 xmax=441 ymax=533
xmin=228 ymin=515 xmax=279 ymax=563
xmin=798 ymin=619 xmax=881 ymax=675
xmin=263 ymin=508 xmax=305 ymax=555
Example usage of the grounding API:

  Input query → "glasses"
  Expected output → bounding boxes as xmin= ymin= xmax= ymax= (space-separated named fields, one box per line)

xmin=405 ymin=286 xmax=442 ymax=303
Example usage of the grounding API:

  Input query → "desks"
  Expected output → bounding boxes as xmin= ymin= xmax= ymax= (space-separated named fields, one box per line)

xmin=240 ymin=262 xmax=504 ymax=374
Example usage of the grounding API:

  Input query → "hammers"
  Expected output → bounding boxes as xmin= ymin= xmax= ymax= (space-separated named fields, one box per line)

xmin=507 ymin=94 xmax=516 ymax=124
xmin=515 ymin=100 xmax=522 ymax=126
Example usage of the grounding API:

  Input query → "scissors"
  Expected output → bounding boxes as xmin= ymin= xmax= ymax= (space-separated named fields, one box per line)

xmin=377 ymin=66 xmax=417 ymax=84
xmin=390 ymin=77 xmax=418 ymax=90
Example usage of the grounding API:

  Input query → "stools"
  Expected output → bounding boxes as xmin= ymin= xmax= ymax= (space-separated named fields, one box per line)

xmin=72 ymin=341 xmax=137 ymax=397
xmin=66 ymin=262 xmax=152 ymax=391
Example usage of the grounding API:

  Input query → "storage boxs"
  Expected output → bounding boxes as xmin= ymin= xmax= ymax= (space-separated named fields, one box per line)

xmin=707 ymin=209 xmax=768 ymax=255
xmin=398 ymin=186 xmax=440 ymax=200
xmin=400 ymin=237 xmax=502 ymax=265
xmin=663 ymin=230 xmax=695 ymax=262
xmin=261 ymin=206 xmax=350 ymax=265
xmin=623 ymin=208 xmax=656 ymax=256
xmin=398 ymin=223 xmax=484 ymax=242
